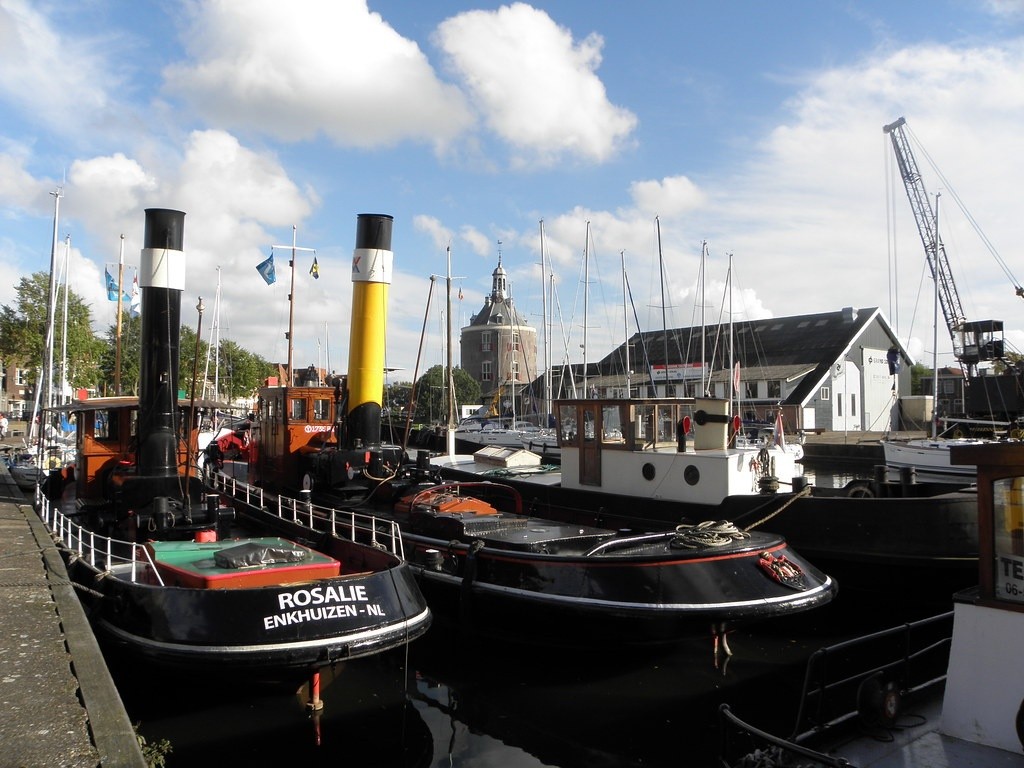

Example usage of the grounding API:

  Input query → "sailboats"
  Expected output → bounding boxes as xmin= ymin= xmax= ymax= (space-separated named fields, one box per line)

xmin=0 ymin=183 xmax=1024 ymax=741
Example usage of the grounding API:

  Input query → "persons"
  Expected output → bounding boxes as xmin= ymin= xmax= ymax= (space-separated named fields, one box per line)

xmin=644 ymin=439 xmax=656 ymax=451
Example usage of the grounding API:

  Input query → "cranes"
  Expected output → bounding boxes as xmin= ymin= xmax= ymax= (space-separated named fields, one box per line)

xmin=883 ymin=116 xmax=1024 ymax=387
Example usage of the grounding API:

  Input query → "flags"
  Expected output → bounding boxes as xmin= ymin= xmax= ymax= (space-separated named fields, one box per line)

xmin=459 ymin=287 xmax=463 ymax=299
xmin=132 ymin=268 xmax=139 ymax=298
xmin=309 ymin=255 xmax=319 ymax=279
xmin=774 ymin=409 xmax=787 ymax=453
xmin=255 ymin=251 xmax=277 ymax=286
xmin=104 ymin=268 xmax=130 ymax=302
